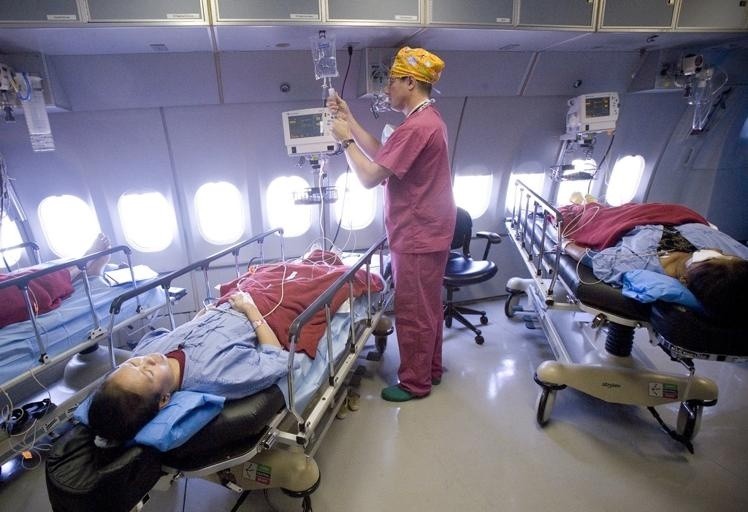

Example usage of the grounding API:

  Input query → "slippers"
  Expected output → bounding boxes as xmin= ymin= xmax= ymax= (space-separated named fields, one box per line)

xmin=345 ymin=384 xmax=361 ymax=411
xmin=333 ymin=393 xmax=349 ymax=420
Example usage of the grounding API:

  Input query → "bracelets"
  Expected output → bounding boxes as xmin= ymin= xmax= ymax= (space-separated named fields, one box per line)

xmin=341 ymin=138 xmax=355 ymax=151
xmin=252 ymin=319 xmax=266 ymax=331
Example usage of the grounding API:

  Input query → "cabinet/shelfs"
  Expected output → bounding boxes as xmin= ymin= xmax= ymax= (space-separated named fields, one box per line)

xmin=210 ymin=2 xmax=426 ymax=25
xmin=426 ymin=0 xmax=602 ymax=34
xmin=598 ymin=2 xmax=679 ymax=31
xmin=0 ymin=0 xmax=206 ymax=26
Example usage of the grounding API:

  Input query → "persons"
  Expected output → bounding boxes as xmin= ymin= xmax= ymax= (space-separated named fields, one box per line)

xmin=0 ymin=232 xmax=113 ymax=331
xmin=320 ymin=43 xmax=459 ymax=402
xmin=85 ymin=246 xmax=389 ymax=462
xmin=548 ymin=191 xmax=747 ymax=328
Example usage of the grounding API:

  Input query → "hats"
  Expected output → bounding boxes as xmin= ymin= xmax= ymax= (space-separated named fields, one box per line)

xmin=389 ymin=45 xmax=446 ymax=87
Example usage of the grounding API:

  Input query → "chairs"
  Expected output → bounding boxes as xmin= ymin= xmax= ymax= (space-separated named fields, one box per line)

xmin=434 ymin=207 xmax=504 ymax=344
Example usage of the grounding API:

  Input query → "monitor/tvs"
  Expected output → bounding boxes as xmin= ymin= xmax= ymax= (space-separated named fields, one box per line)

xmin=281 ymin=107 xmax=339 ymax=154
xmin=565 ymin=91 xmax=620 ymax=133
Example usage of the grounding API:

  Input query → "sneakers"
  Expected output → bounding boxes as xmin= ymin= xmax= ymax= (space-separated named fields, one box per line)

xmin=381 ymin=377 xmax=442 ymax=402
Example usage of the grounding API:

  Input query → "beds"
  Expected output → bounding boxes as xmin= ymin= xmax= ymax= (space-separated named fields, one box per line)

xmin=499 ymin=186 xmax=748 ymax=441
